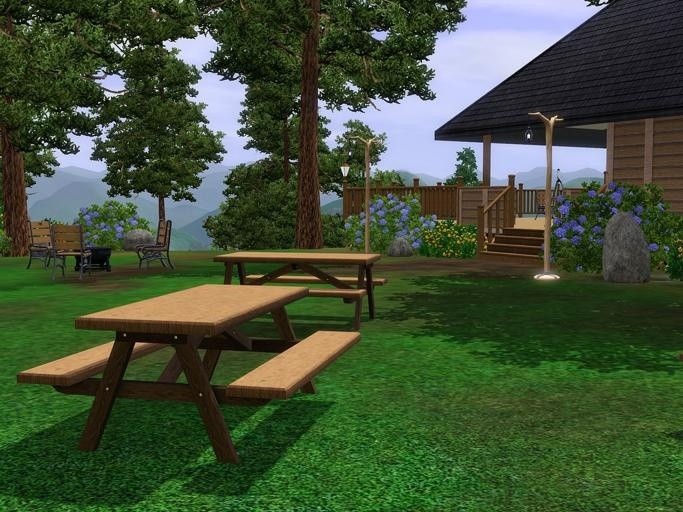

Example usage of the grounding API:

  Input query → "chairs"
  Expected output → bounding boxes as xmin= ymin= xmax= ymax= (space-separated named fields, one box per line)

xmin=534 ymin=184 xmax=561 ymax=220
xmin=26 ymin=218 xmax=175 ymax=281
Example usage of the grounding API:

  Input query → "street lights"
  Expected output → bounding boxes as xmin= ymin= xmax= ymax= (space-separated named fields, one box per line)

xmin=340 ymin=135 xmax=378 ymax=254
xmin=522 ymin=111 xmax=563 ymax=274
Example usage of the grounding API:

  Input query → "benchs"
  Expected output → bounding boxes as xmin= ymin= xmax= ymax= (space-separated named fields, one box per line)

xmin=16 ymin=274 xmax=387 ymax=407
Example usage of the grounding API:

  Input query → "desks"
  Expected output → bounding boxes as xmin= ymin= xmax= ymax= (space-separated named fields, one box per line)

xmin=75 ymin=283 xmax=310 ymax=464
xmin=213 ymin=251 xmax=381 ymax=330
